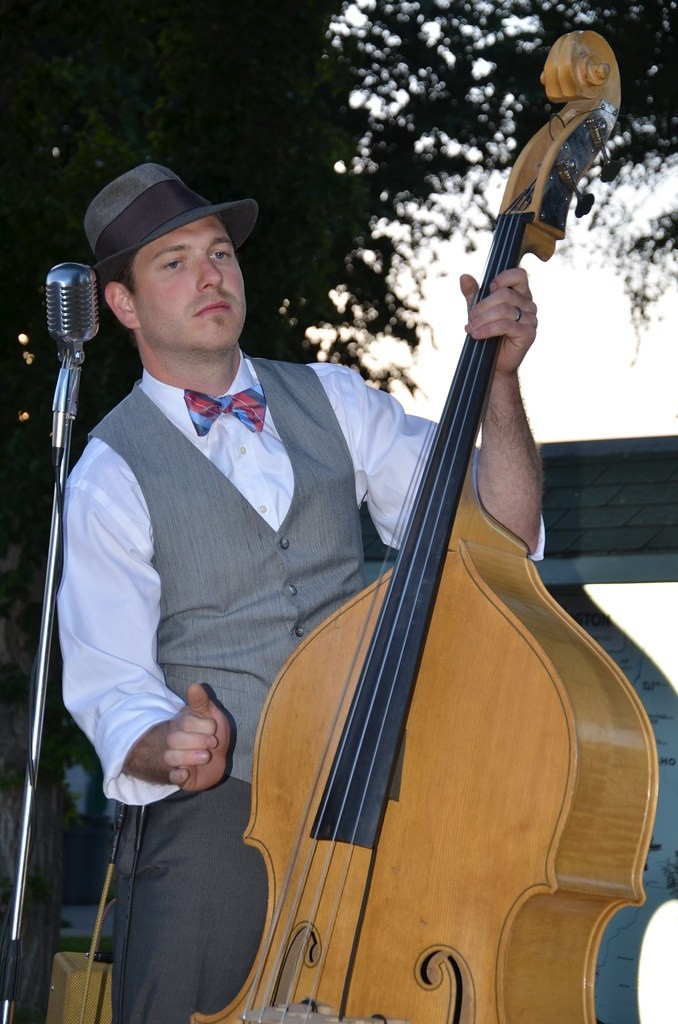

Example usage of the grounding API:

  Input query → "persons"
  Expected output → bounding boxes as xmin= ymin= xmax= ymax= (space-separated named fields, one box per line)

xmin=56 ymin=163 xmax=545 ymax=1023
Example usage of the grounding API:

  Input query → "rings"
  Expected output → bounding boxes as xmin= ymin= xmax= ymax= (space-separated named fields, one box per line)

xmin=513 ymin=306 xmax=522 ymax=322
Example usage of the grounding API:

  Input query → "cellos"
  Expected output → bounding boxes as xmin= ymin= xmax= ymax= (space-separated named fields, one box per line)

xmin=189 ymin=30 xmax=660 ymax=1024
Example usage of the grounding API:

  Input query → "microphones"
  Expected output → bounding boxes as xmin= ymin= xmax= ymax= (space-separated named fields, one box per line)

xmin=46 ymin=262 xmax=100 ymax=467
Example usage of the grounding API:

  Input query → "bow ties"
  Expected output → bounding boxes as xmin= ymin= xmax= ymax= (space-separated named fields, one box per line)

xmin=184 ymin=384 xmax=266 ymax=436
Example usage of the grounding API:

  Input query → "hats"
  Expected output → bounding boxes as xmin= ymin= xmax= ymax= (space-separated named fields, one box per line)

xmin=84 ymin=162 xmax=258 ymax=306
